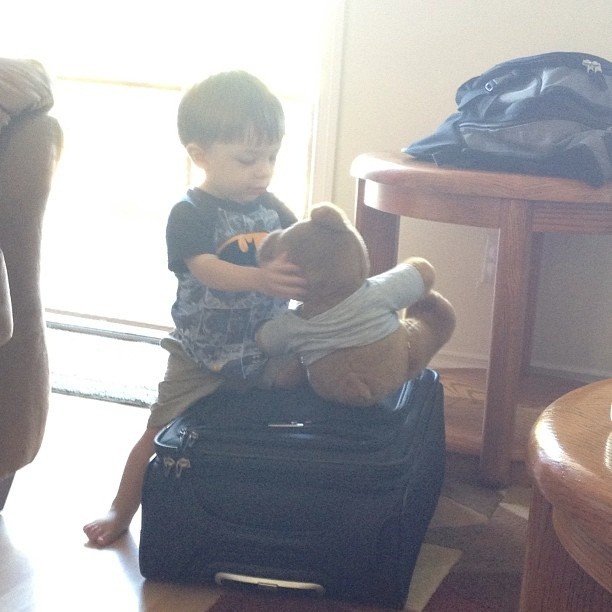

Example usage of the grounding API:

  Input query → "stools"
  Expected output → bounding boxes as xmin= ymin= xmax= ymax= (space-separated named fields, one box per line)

xmin=520 ymin=379 xmax=612 ymax=611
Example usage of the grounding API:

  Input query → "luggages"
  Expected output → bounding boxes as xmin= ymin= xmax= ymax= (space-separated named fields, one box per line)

xmin=138 ymin=368 xmax=445 ymax=608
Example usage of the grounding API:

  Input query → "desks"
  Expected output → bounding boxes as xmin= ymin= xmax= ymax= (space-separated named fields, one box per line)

xmin=350 ymin=150 xmax=611 ymax=488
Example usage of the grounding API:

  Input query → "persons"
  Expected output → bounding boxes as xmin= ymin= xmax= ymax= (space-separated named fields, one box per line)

xmin=83 ymin=67 xmax=307 ymax=547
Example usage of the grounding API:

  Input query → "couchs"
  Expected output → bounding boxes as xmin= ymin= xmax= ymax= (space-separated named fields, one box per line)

xmin=0 ymin=54 xmax=64 ymax=507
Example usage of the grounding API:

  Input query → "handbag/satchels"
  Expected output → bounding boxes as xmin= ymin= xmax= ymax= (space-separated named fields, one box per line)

xmin=400 ymin=52 xmax=611 ymax=187
